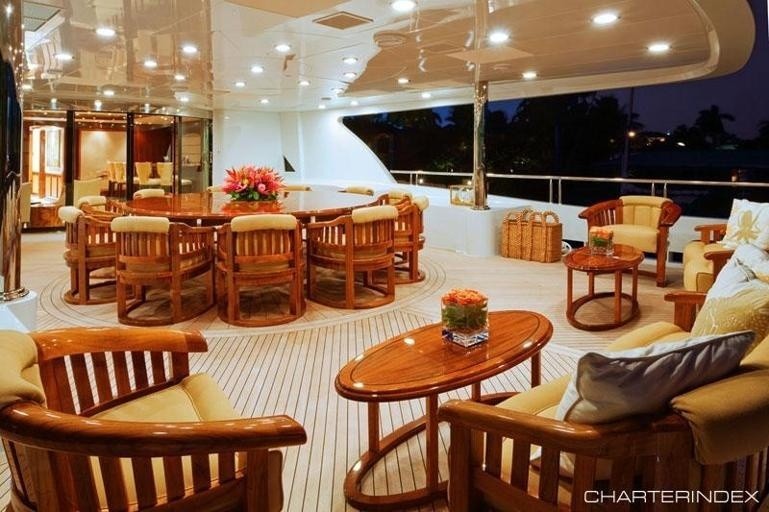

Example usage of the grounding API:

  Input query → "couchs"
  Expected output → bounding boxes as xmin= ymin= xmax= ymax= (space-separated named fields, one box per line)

xmin=436 ymin=289 xmax=769 ymax=511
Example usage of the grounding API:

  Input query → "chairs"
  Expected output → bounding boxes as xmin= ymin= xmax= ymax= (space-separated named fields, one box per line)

xmin=680 ymin=223 xmax=735 ymax=290
xmin=0 ymin=322 xmax=308 ymax=511
xmin=575 ymin=194 xmax=677 ymax=289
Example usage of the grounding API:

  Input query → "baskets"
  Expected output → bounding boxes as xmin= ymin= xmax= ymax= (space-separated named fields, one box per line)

xmin=501 ymin=209 xmax=562 ymax=263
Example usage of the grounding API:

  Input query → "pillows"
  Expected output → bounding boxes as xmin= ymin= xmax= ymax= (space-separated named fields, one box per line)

xmin=684 ymin=253 xmax=768 ymax=360
xmin=720 ymin=199 xmax=769 ymax=252
xmin=731 ymin=238 xmax=767 ymax=281
xmin=530 ymin=328 xmax=755 ymax=484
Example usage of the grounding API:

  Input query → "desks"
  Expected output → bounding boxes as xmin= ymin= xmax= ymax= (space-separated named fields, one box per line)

xmin=333 ymin=308 xmax=555 ymax=511
xmin=563 ymin=244 xmax=645 ymax=331
